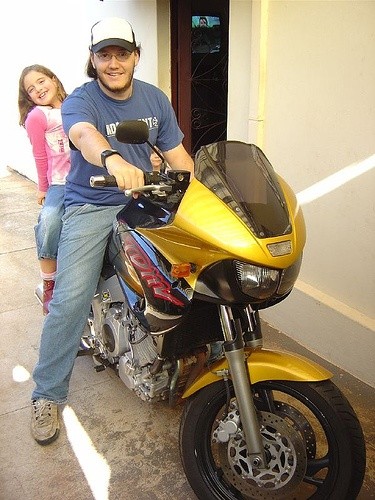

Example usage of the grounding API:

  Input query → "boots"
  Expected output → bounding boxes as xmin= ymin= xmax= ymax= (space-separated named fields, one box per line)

xmin=42 ymin=279 xmax=55 ymax=315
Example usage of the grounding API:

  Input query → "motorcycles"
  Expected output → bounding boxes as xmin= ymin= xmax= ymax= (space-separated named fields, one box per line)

xmin=31 ymin=119 xmax=368 ymax=500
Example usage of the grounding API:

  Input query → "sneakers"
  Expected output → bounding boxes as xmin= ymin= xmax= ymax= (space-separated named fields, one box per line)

xmin=31 ymin=398 xmax=61 ymax=446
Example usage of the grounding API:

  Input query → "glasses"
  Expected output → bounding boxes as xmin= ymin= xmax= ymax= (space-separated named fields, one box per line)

xmin=94 ymin=52 xmax=131 ymax=62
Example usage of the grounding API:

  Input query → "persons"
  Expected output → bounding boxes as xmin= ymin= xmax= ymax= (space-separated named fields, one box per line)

xmin=19 ymin=65 xmax=73 ymax=313
xmin=197 ymin=17 xmax=208 ymax=29
xmin=150 ymin=145 xmax=164 ymax=172
xmin=31 ymin=17 xmax=195 ymax=443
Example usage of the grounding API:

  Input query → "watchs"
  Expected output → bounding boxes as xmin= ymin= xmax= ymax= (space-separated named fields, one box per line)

xmin=101 ymin=149 xmax=123 ymax=170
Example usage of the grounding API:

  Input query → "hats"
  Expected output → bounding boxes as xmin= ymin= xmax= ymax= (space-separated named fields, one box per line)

xmin=88 ymin=17 xmax=136 ymax=53
